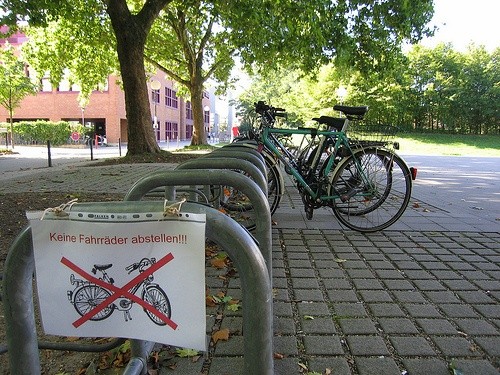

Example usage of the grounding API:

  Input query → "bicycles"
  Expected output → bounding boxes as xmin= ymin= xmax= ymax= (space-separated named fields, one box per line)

xmin=211 ymin=100 xmax=418 ymax=234
